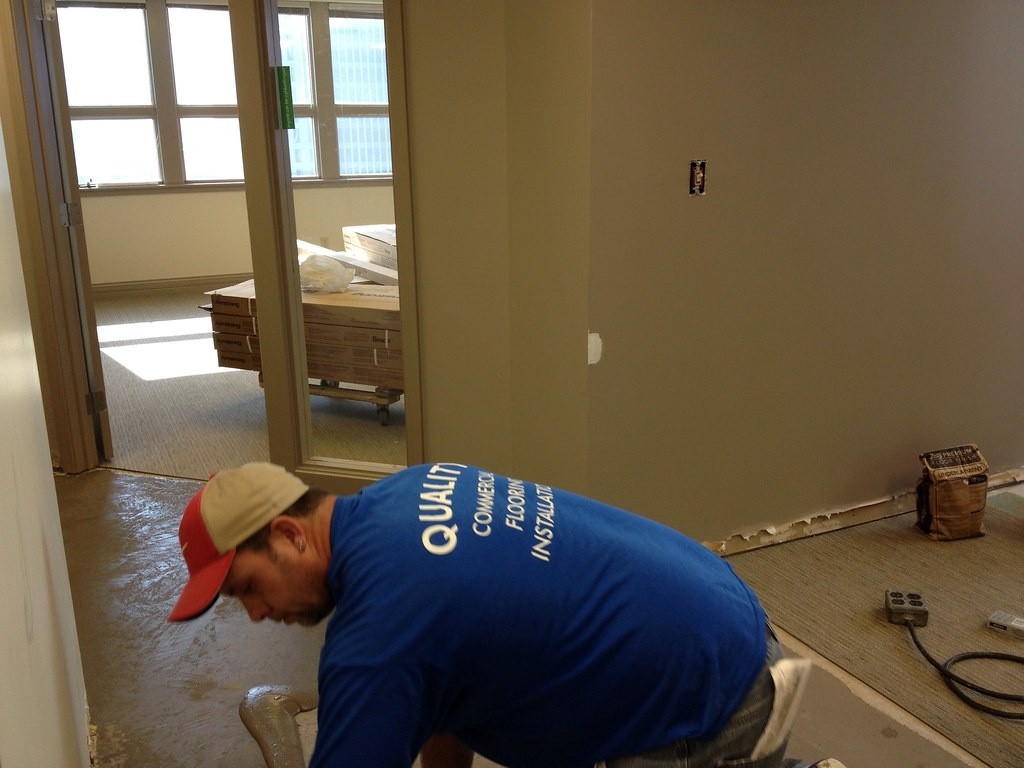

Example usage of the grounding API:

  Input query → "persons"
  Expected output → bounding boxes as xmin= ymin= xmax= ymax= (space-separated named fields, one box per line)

xmin=160 ymin=460 xmax=849 ymax=767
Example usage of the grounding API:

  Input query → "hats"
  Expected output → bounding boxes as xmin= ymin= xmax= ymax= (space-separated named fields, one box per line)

xmin=167 ymin=462 xmax=309 ymax=623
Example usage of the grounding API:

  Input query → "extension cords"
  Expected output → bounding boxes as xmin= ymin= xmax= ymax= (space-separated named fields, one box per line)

xmin=885 ymin=589 xmax=929 ymax=627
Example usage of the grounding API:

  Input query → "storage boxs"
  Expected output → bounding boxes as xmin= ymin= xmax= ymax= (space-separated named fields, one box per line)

xmin=344 ymin=241 xmax=397 ymax=271
xmin=210 ymin=312 xmax=402 ymax=351
xmin=212 ymin=332 xmax=403 ymax=369
xmin=218 ymin=351 xmax=403 ymax=389
xmin=199 ymin=279 xmax=401 ymax=331
xmin=342 ymin=223 xmax=397 ymax=262
